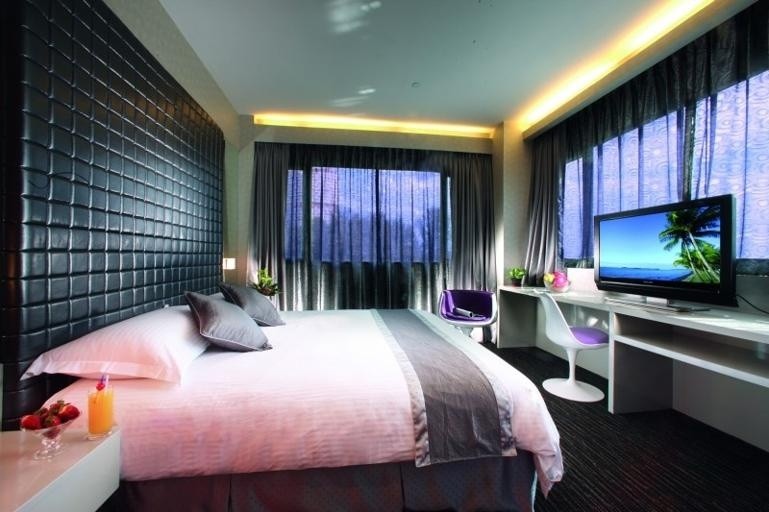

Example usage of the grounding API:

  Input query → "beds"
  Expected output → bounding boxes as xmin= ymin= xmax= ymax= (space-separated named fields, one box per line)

xmin=43 ymin=308 xmax=564 ymax=510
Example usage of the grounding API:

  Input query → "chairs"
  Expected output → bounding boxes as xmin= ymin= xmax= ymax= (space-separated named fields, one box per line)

xmin=537 ymin=294 xmax=608 ymax=402
xmin=440 ymin=289 xmax=498 ymax=342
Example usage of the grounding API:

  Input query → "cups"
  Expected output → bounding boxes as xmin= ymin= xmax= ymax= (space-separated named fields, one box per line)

xmin=87 ymin=384 xmax=113 ymax=440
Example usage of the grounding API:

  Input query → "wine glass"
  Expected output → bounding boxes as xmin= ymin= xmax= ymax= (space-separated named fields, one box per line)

xmin=20 ymin=412 xmax=82 ymax=461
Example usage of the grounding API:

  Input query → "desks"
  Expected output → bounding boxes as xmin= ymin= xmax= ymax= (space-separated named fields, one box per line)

xmin=610 ymin=292 xmax=768 ymax=461
xmin=499 ymin=283 xmax=613 ymax=412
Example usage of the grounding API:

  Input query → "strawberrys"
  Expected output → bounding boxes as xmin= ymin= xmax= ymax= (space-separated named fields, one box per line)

xmin=21 ymin=400 xmax=80 ymax=438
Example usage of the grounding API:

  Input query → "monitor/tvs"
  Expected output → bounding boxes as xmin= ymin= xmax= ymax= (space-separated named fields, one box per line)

xmin=594 ymin=194 xmax=738 ymax=311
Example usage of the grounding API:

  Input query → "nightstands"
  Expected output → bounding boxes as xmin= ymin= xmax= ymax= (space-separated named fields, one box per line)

xmin=0 ymin=421 xmax=123 ymax=510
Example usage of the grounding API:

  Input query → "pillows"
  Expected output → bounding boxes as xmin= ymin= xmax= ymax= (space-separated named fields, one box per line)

xmin=17 ymin=304 xmax=212 ymax=384
xmin=184 ymin=288 xmax=272 ymax=353
xmin=220 ymin=285 xmax=286 ymax=327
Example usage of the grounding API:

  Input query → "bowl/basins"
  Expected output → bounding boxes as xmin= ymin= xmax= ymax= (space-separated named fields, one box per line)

xmin=542 ymin=280 xmax=571 ymax=293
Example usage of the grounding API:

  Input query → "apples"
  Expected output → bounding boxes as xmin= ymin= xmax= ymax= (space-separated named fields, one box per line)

xmin=544 ymin=272 xmax=555 ymax=284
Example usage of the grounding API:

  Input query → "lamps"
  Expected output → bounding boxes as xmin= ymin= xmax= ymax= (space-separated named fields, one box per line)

xmin=221 ymin=257 xmax=238 ymax=283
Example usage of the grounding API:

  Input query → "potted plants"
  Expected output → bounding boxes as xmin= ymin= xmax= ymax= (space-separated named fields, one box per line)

xmin=509 ymin=266 xmax=526 ymax=286
